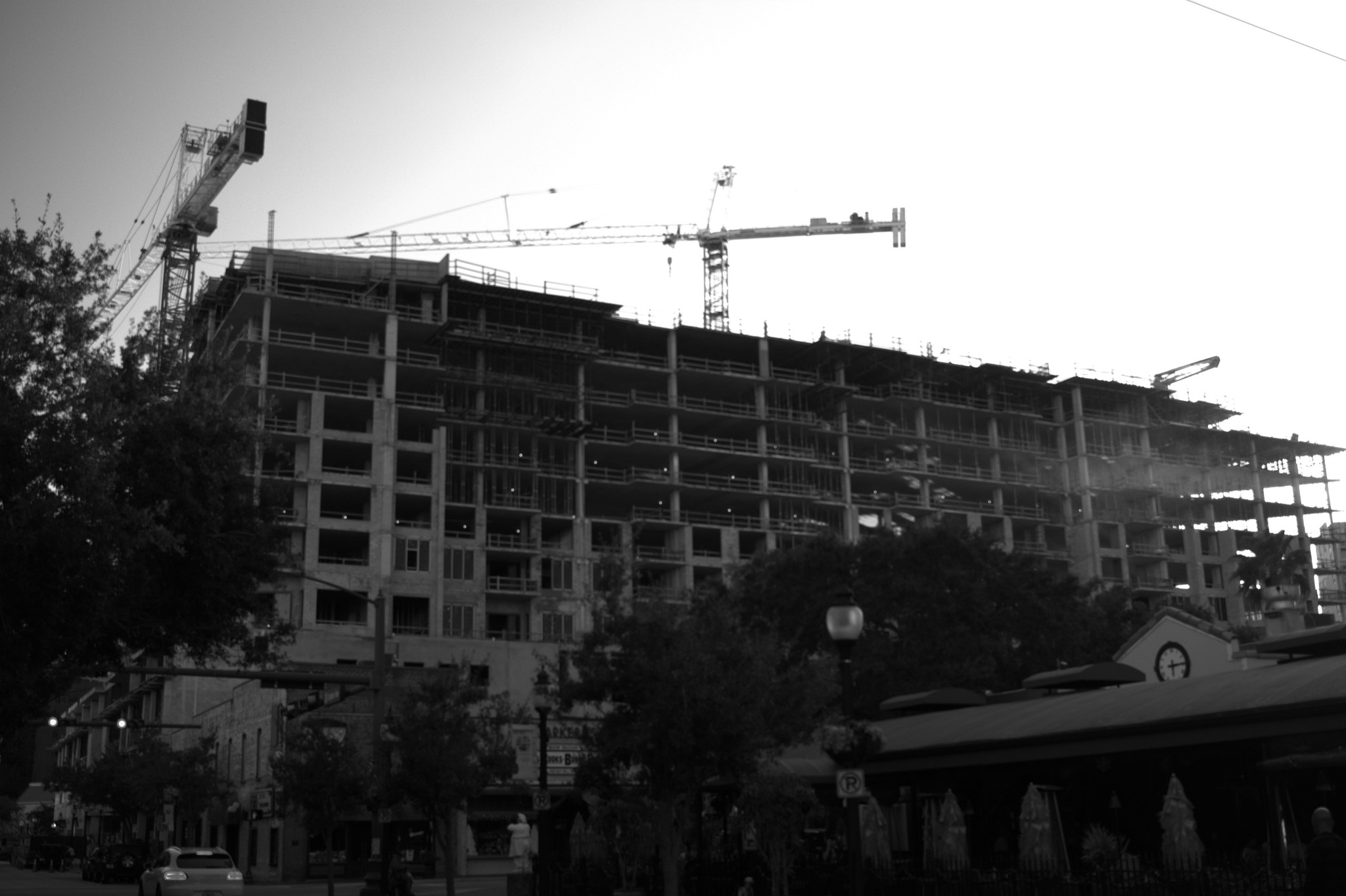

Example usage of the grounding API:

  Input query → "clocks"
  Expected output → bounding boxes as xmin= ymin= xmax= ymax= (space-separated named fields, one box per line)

xmin=1154 ymin=641 xmax=1190 ymax=682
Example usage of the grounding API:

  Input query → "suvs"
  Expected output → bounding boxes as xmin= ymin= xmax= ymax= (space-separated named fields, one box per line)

xmin=86 ymin=843 xmax=145 ymax=884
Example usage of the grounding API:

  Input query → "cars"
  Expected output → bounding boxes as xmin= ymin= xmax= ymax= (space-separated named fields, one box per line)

xmin=139 ymin=846 xmax=244 ymax=896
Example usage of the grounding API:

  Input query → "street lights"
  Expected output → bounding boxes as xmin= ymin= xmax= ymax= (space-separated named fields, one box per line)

xmin=324 ymin=715 xmax=347 ymax=896
xmin=532 ymin=665 xmax=553 ymax=896
xmin=98 ymin=803 xmax=105 ymax=844
xmin=26 ymin=817 xmax=30 ymax=838
xmin=84 ymin=806 xmax=89 ymax=837
xmin=71 ymin=804 xmax=77 ymax=837
xmin=58 ymin=812 xmax=63 ymax=839
xmin=827 ymin=586 xmax=864 ymax=896
xmin=32 ymin=816 xmax=38 ymax=837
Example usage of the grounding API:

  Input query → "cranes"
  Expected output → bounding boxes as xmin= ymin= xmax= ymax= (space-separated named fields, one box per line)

xmin=61 ymin=99 xmax=906 ymax=406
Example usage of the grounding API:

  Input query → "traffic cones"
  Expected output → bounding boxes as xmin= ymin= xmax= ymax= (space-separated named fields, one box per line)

xmin=49 ymin=858 xmax=55 ymax=873
xmin=58 ymin=859 xmax=66 ymax=873
xmin=32 ymin=858 xmax=38 ymax=872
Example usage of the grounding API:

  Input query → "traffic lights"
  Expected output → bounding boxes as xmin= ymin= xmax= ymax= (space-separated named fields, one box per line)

xmin=48 ymin=717 xmax=77 ymax=727
xmin=117 ymin=719 xmax=145 ymax=728
